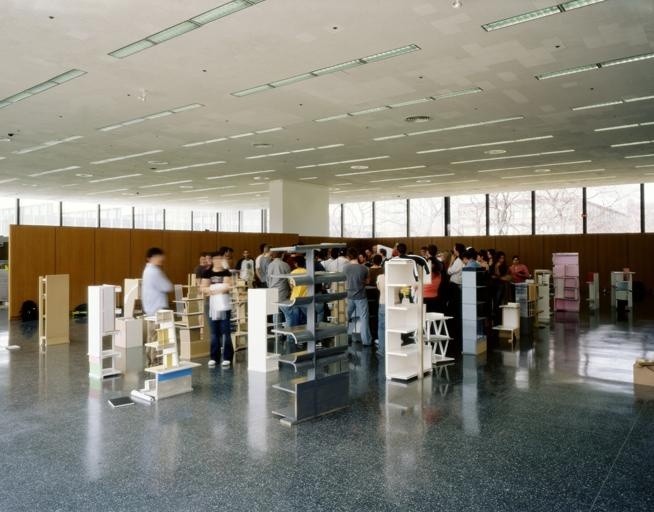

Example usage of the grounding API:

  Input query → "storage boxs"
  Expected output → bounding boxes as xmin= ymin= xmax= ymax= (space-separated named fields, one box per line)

xmin=632 ymin=357 xmax=653 ymax=385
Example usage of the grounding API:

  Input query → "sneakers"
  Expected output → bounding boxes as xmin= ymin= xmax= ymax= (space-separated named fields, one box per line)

xmin=222 ymin=361 xmax=230 ymax=366
xmin=208 ymin=360 xmax=215 ymax=366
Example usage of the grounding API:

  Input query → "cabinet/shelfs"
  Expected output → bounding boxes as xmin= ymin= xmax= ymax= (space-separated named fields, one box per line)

xmin=611 ymin=272 xmax=635 ymax=309
xmin=87 ymin=268 xmax=279 ymax=404
xmin=513 ymin=283 xmax=547 ymax=330
xmin=533 ymin=267 xmax=552 ymax=323
xmin=552 ymin=252 xmax=580 ymax=312
xmin=586 ymin=273 xmax=600 ymax=310
xmin=460 ymin=266 xmax=487 ymax=356
xmin=420 ymin=310 xmax=457 ymax=365
xmin=268 ymin=242 xmax=350 ymax=427
xmin=384 ymin=259 xmax=432 ymax=383
xmin=38 ymin=274 xmax=70 ymax=344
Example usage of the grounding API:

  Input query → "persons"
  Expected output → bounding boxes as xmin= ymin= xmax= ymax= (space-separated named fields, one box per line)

xmin=196 ymin=243 xmax=531 ymax=366
xmin=140 ymin=247 xmax=174 ymax=364
xmin=209 ymin=365 xmax=230 ymax=370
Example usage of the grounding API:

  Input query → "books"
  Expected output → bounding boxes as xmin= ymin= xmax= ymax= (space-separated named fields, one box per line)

xmin=615 ymin=281 xmax=629 ymax=301
